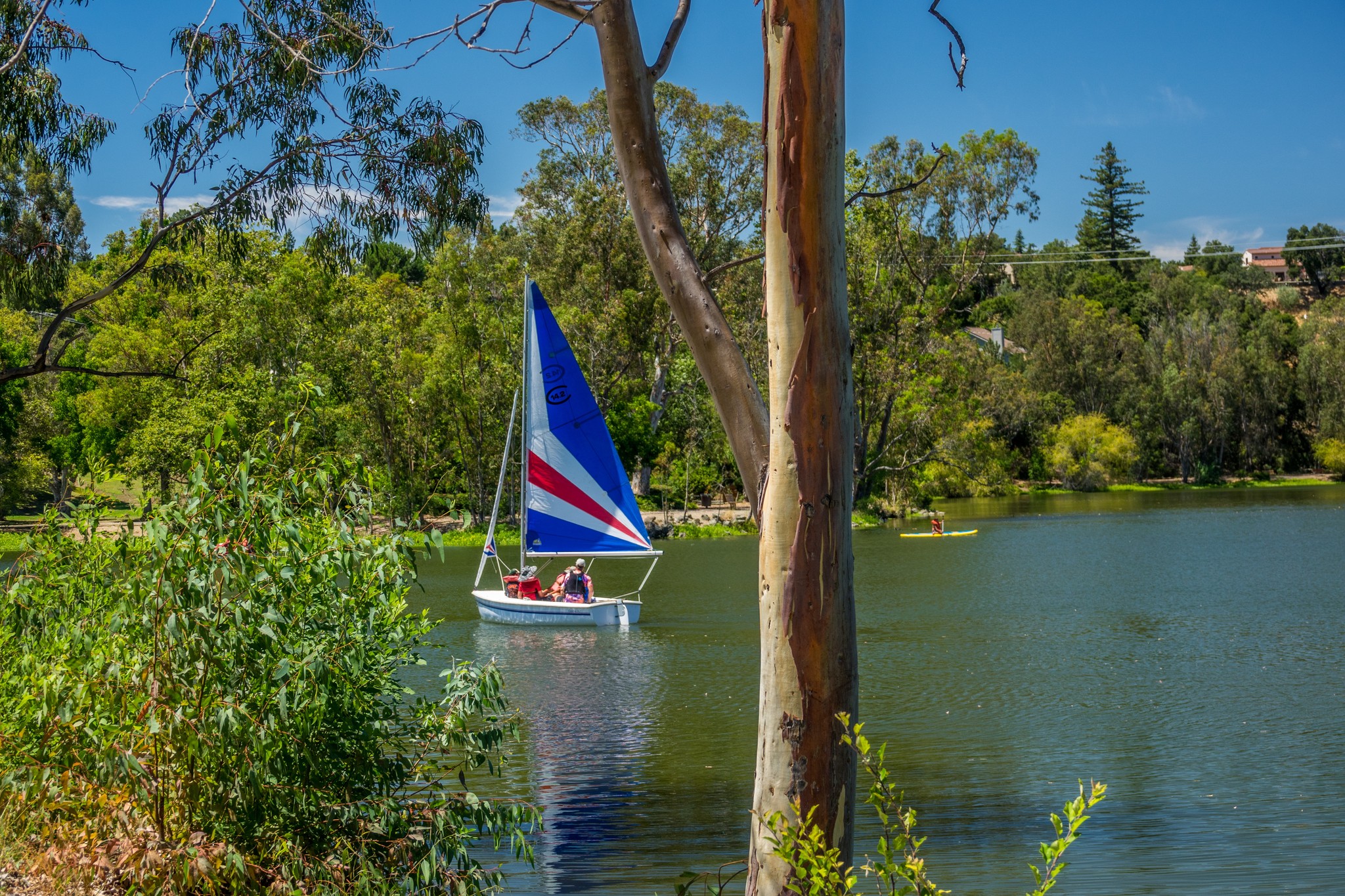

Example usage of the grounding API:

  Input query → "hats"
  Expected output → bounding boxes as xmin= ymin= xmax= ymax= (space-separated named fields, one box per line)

xmin=576 ymin=559 xmax=585 ymax=568
xmin=509 ymin=569 xmax=520 ymax=575
xmin=564 ymin=566 xmax=576 ymax=573
xmin=518 ymin=565 xmax=537 ymax=582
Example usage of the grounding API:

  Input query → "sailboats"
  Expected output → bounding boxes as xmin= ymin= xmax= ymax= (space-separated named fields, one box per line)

xmin=471 ymin=273 xmax=664 ymax=626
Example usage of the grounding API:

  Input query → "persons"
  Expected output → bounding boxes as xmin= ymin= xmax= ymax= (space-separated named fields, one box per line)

xmin=505 ymin=558 xmax=594 ymax=604
xmin=932 ymin=517 xmax=944 ymax=534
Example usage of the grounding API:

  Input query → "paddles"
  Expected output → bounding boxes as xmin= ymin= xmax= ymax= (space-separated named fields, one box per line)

xmin=940 ymin=511 xmax=946 ymax=537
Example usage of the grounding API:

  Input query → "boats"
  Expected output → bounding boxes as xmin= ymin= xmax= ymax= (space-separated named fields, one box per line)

xmin=900 ymin=529 xmax=979 ymax=538
xmin=212 ymin=542 xmax=280 ymax=560
